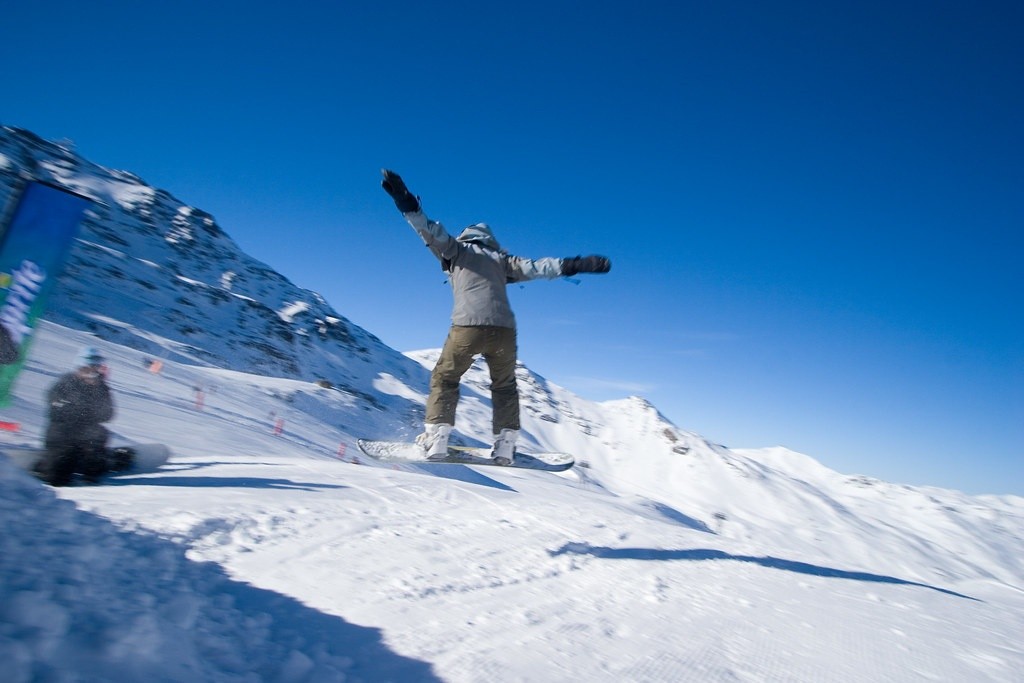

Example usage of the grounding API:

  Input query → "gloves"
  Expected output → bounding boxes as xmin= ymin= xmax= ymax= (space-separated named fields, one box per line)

xmin=562 ymin=256 xmax=612 ymax=276
xmin=380 ymin=169 xmax=419 ymax=212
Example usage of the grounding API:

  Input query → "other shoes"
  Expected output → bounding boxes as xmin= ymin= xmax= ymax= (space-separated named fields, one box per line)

xmin=417 ymin=425 xmax=451 ymax=460
xmin=491 ymin=428 xmax=517 ymax=466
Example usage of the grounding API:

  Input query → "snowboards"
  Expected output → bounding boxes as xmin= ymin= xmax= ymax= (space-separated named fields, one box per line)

xmin=356 ymin=437 xmax=576 ymax=472
xmin=29 ymin=443 xmax=169 ymax=482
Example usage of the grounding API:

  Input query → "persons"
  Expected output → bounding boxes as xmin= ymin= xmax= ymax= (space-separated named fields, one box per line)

xmin=381 ymin=169 xmax=612 ymax=466
xmin=43 ymin=348 xmax=112 ymax=484
